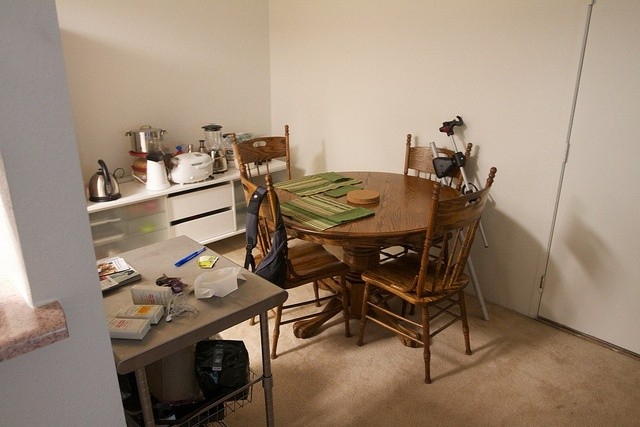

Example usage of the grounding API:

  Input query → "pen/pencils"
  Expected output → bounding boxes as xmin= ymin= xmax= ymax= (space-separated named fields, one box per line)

xmin=175 ymin=246 xmax=207 ymax=266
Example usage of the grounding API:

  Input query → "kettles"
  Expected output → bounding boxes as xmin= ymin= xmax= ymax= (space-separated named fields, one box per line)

xmin=89 ymin=159 xmax=122 ymax=203
xmin=145 ymin=153 xmax=170 ymax=190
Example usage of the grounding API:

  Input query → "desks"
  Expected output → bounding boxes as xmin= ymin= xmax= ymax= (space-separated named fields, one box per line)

xmin=97 ymin=235 xmax=288 ymax=427
xmin=258 ymin=171 xmax=470 ymax=348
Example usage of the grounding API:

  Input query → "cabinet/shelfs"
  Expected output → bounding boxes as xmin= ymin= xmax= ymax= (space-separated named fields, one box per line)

xmin=88 ymin=195 xmax=170 ymax=259
xmin=167 ymin=180 xmax=235 ymax=244
xmin=235 ymin=167 xmax=288 ymax=231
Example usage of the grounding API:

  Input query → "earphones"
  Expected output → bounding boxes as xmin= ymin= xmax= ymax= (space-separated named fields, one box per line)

xmin=165 ymin=313 xmax=173 ymax=322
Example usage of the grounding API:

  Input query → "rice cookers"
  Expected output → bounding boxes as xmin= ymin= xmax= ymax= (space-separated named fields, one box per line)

xmin=166 ymin=152 xmax=215 ymax=186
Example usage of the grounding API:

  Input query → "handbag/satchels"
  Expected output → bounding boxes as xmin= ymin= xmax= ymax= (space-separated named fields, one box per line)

xmin=244 ymin=185 xmax=288 ymax=287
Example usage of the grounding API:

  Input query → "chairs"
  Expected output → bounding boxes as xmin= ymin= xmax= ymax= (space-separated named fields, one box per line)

xmin=231 ymin=125 xmax=310 ymax=251
xmin=357 ymin=166 xmax=497 ymax=383
xmin=378 ymin=133 xmax=473 ymax=321
xmin=239 ymin=164 xmax=351 ymax=358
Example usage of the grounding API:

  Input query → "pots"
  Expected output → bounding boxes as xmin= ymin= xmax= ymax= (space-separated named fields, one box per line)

xmin=125 ymin=124 xmax=166 ymax=152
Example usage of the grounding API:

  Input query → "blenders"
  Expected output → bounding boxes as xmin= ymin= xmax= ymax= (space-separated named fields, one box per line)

xmin=200 ymin=125 xmax=228 ymax=174
